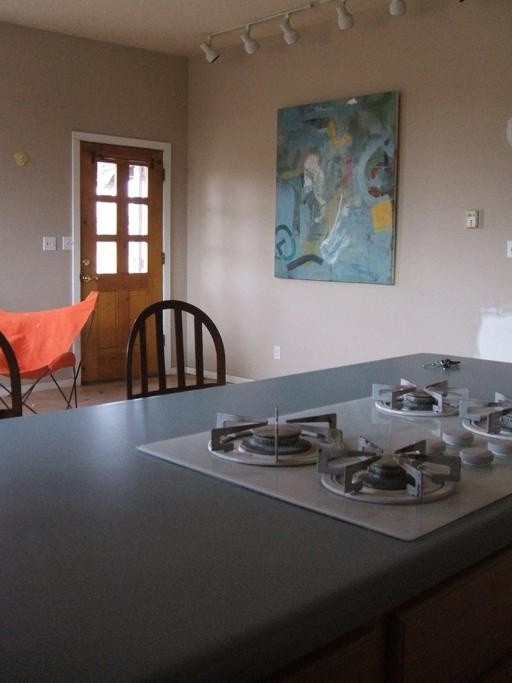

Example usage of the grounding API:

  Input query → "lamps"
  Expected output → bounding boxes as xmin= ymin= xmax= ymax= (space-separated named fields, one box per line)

xmin=199 ymin=3 xmax=417 ymax=62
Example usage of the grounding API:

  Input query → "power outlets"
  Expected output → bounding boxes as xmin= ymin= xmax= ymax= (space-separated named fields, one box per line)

xmin=43 ymin=237 xmax=57 ymax=251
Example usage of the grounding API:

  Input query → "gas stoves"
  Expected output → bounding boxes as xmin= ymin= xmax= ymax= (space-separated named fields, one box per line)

xmin=135 ymin=376 xmax=510 ymax=555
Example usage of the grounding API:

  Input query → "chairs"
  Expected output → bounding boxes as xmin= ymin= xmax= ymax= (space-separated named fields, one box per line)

xmin=0 ymin=291 xmax=100 ymax=414
xmin=125 ymin=302 xmax=225 ymax=395
